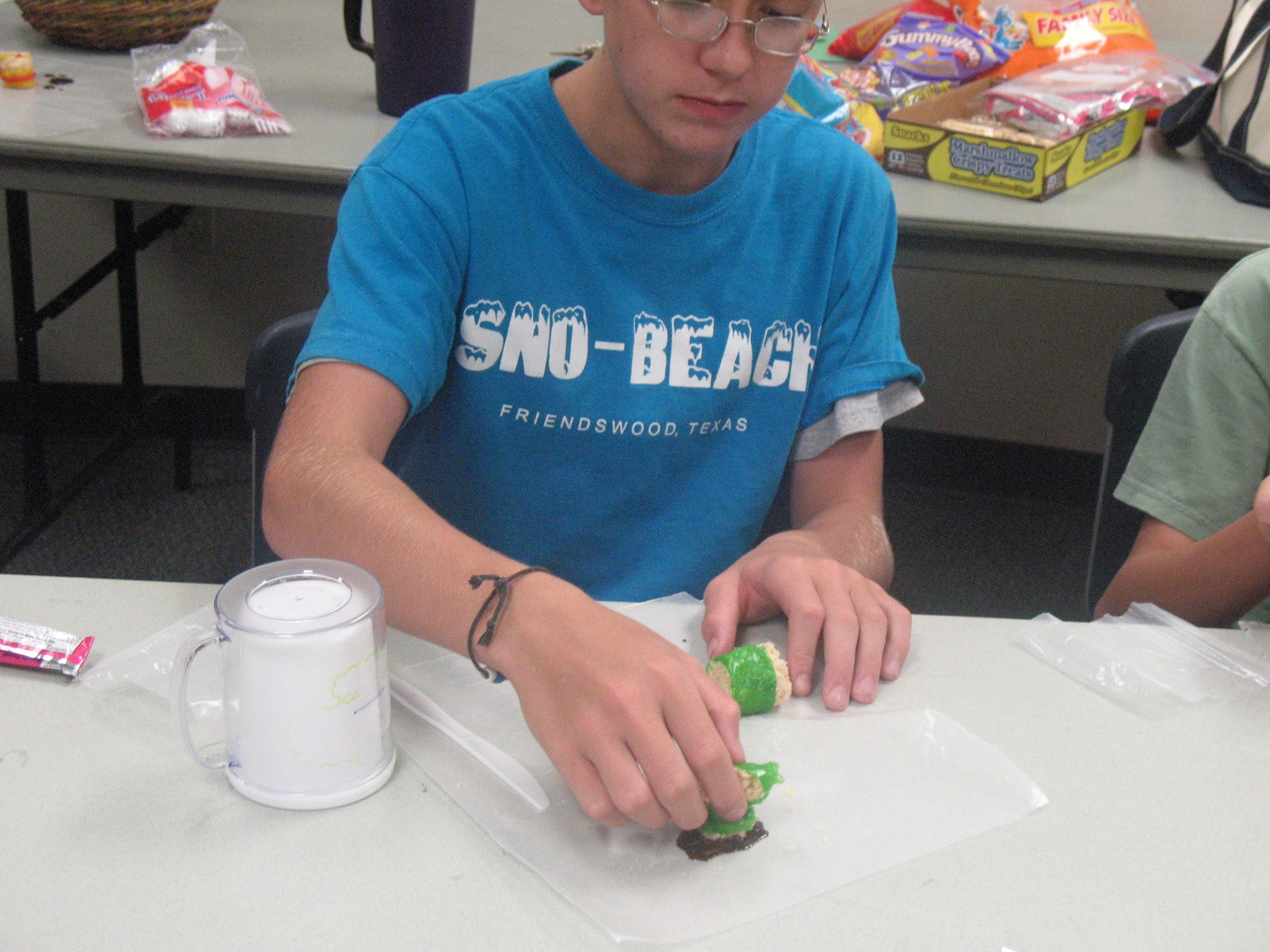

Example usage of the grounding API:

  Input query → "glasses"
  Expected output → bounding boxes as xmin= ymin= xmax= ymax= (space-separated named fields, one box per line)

xmin=648 ymin=0 xmax=830 ymax=58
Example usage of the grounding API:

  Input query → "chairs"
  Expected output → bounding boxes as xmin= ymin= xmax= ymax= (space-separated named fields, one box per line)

xmin=1083 ymin=306 xmax=1205 ymax=624
xmin=242 ymin=308 xmax=322 ymax=566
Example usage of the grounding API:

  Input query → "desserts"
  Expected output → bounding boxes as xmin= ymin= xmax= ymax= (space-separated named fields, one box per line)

xmin=708 ymin=640 xmax=791 ymax=716
xmin=0 ymin=51 xmax=36 ymax=89
xmin=696 ymin=761 xmax=780 ymax=843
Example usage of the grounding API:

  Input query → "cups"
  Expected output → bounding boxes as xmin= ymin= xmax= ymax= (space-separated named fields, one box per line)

xmin=171 ymin=558 xmax=396 ymax=809
xmin=342 ymin=0 xmax=475 ymax=118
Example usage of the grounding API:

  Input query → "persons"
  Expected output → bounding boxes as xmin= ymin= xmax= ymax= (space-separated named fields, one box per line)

xmin=1092 ymin=247 xmax=1270 ymax=628
xmin=260 ymin=0 xmax=928 ymax=832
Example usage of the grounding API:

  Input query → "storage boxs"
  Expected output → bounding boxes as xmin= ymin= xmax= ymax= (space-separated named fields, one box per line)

xmin=883 ymin=76 xmax=1153 ymax=201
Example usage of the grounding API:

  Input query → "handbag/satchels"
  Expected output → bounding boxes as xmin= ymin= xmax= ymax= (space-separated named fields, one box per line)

xmin=1158 ymin=0 xmax=1270 ymax=210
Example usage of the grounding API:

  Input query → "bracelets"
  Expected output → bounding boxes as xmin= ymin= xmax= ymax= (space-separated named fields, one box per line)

xmin=466 ymin=562 xmax=558 ymax=689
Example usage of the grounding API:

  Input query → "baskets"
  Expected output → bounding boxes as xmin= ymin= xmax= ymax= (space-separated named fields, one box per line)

xmin=14 ymin=0 xmax=217 ymax=50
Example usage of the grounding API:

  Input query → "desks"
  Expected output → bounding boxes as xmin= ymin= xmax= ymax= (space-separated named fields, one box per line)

xmin=0 ymin=573 xmax=1270 ymax=952
xmin=0 ymin=20 xmax=1270 ymax=578
